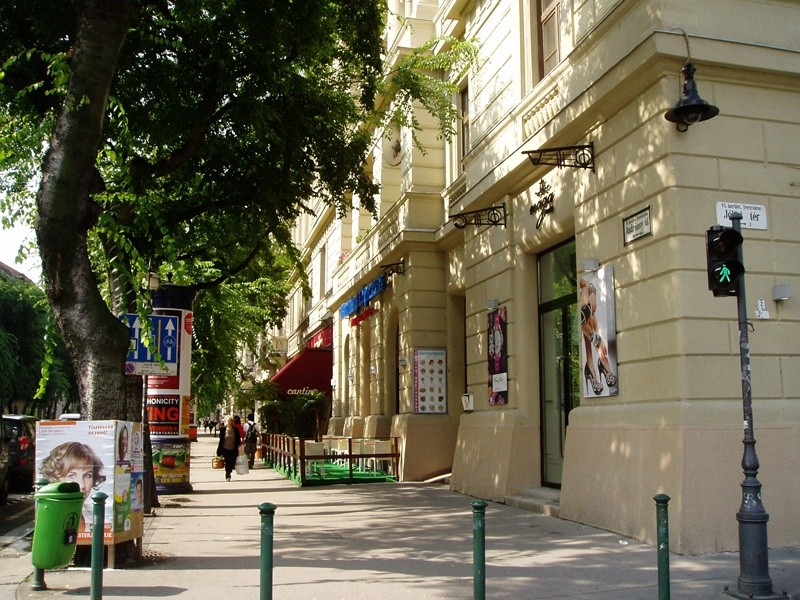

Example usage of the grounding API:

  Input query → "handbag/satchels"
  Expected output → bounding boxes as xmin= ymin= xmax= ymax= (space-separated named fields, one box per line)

xmin=234 ymin=445 xmax=249 ymax=475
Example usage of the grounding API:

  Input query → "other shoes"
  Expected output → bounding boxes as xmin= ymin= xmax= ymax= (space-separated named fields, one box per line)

xmin=227 ymin=477 xmax=230 ymax=482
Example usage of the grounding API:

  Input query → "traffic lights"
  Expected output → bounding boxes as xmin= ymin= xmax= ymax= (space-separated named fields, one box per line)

xmin=704 ymin=224 xmax=745 ymax=298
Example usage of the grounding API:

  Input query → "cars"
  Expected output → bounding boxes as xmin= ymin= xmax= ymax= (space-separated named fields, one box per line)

xmin=1 ymin=414 xmax=41 ymax=491
xmin=58 ymin=413 xmax=81 ymax=421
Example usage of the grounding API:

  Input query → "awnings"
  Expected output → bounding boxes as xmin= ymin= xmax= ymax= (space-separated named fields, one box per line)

xmin=256 ymin=349 xmax=333 ymax=401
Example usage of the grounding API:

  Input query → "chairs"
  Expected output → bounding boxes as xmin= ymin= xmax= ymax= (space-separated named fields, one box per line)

xmin=305 ymin=435 xmax=392 ymax=479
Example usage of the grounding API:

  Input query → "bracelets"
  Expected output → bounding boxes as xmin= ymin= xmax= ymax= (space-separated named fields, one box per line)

xmin=588 ymin=329 xmax=602 ymax=348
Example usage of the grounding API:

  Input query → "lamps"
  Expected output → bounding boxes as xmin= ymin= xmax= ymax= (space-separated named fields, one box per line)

xmin=665 ymin=29 xmax=720 ymax=125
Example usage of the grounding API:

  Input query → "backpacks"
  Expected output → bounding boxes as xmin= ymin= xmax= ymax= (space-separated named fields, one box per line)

xmin=245 ymin=423 xmax=257 ymax=444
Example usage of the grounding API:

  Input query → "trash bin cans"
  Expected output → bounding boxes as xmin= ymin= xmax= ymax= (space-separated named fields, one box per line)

xmin=31 ymin=481 xmax=84 ymax=569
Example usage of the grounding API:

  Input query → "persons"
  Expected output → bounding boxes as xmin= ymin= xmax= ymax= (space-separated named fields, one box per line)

xmin=39 ymin=425 xmax=142 ymax=532
xmin=197 ymin=414 xmax=261 ymax=481
xmin=579 ymin=277 xmax=616 ymax=395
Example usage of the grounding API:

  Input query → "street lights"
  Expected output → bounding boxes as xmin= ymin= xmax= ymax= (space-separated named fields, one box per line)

xmin=138 ymin=261 xmax=160 ymax=513
